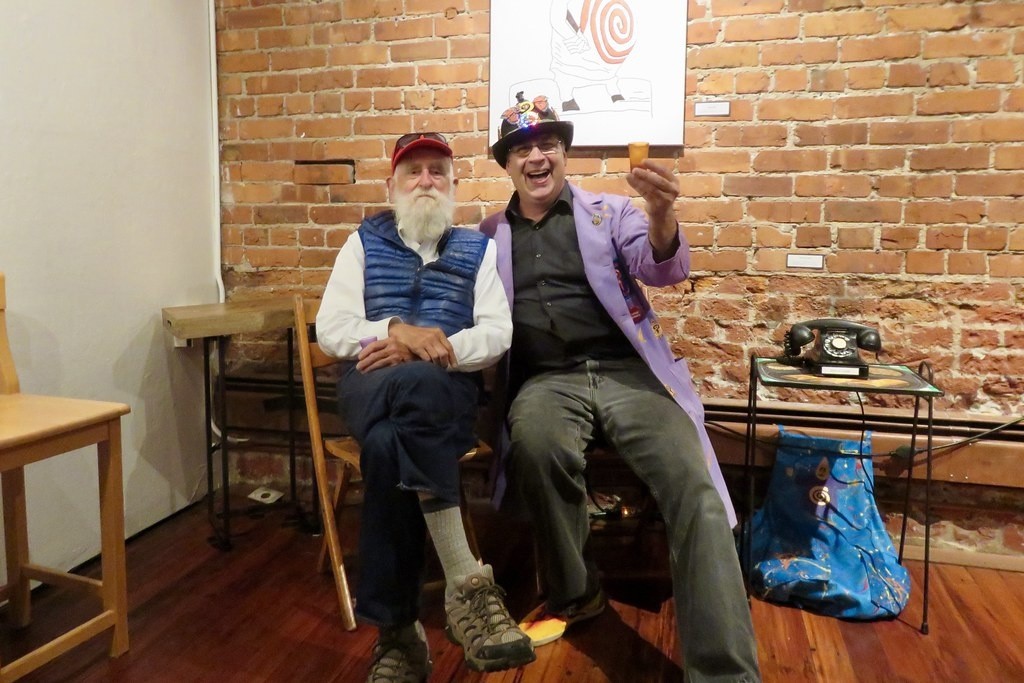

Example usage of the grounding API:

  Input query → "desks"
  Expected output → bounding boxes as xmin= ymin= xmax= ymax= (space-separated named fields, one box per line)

xmin=740 ymin=352 xmax=946 ymax=635
xmin=163 ymin=296 xmax=321 ymax=553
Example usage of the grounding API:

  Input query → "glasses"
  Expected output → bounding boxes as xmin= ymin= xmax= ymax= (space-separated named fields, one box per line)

xmin=507 ymin=138 xmax=564 ymax=157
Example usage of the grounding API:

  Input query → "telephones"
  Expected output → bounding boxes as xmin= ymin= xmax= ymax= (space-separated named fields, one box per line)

xmin=789 ymin=318 xmax=881 ymax=380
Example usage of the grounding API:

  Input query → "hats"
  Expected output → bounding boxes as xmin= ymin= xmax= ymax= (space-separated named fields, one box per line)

xmin=391 ymin=132 xmax=452 ymax=177
xmin=492 ymin=91 xmax=574 ymax=169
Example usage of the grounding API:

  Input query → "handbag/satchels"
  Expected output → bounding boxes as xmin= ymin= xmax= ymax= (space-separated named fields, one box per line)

xmin=734 ymin=423 xmax=912 ymax=620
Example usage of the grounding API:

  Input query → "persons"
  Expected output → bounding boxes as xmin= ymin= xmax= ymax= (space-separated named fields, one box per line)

xmin=479 ymin=99 xmax=765 ymax=683
xmin=316 ymin=131 xmax=538 ymax=683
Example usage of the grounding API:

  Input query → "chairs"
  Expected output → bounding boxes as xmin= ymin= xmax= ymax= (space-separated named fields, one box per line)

xmin=293 ymin=293 xmax=666 ymax=632
xmin=0 ymin=276 xmax=131 ymax=683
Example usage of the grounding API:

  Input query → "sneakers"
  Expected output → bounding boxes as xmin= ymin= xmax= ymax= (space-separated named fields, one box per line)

xmin=518 ymin=586 xmax=607 ymax=647
xmin=366 ymin=622 xmax=431 ymax=683
xmin=443 ymin=564 xmax=536 ymax=672
